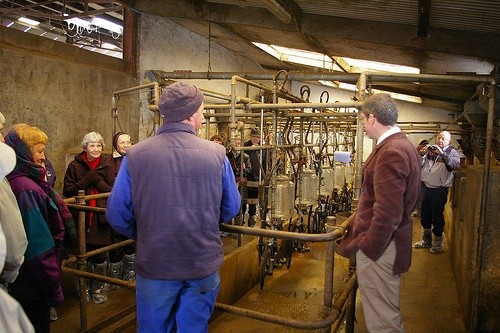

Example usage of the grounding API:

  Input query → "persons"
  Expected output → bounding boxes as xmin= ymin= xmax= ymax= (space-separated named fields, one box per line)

xmin=4 ymin=122 xmax=80 ymax=332
xmin=106 ymin=82 xmax=241 ymax=333
xmin=61 ymin=132 xmax=114 ymax=304
xmin=413 ymin=139 xmax=428 ymax=217
xmin=240 ymin=126 xmax=267 ymax=228
xmin=105 ymin=132 xmax=135 ymax=289
xmin=413 ymin=131 xmax=460 ymax=253
xmin=210 ymin=134 xmax=222 ymax=145
xmin=0 ymin=111 xmax=35 ymax=333
xmin=219 ymin=134 xmax=252 ymax=238
xmin=342 ymin=93 xmax=422 ymax=333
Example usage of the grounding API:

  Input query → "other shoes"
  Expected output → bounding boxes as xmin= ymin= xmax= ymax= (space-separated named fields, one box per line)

xmin=220 ymin=231 xmax=233 ymax=237
xmin=110 ymin=278 xmax=137 ymax=290
xmin=414 ymin=241 xmax=431 ymax=249
xmin=430 ymin=246 xmax=442 ymax=254
xmin=232 ymin=233 xmax=244 ymax=239
xmin=50 ymin=308 xmax=57 ymax=321
xmin=247 ymin=218 xmax=256 ymax=227
xmin=80 ymin=289 xmax=107 ymax=303
xmin=412 ymin=211 xmax=422 ymax=217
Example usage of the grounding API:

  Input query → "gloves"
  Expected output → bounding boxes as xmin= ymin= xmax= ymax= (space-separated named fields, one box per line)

xmin=95 ymin=178 xmax=107 ymax=189
xmin=53 ymin=286 xmax=65 ymax=305
xmin=79 ymin=172 xmax=101 ymax=187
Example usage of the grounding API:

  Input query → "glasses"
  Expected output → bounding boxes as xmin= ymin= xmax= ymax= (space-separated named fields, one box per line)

xmin=358 ymin=114 xmax=369 ymax=121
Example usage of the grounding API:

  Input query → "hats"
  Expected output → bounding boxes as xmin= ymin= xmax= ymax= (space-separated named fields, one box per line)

xmin=249 ymin=127 xmax=261 ymax=136
xmin=419 ymin=140 xmax=429 ymax=146
xmin=157 ymin=83 xmax=203 ymax=123
xmin=112 ymin=132 xmax=128 ymax=150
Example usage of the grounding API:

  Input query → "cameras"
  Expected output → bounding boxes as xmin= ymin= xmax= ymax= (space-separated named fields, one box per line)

xmin=428 ymin=146 xmax=436 ymax=150
